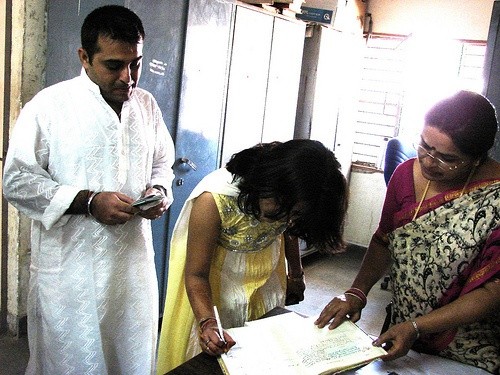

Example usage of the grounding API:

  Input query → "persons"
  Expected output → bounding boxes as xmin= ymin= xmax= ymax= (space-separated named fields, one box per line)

xmin=2 ymin=5 xmax=175 ymax=375
xmin=315 ymin=92 xmax=500 ymax=375
xmin=151 ymin=140 xmax=351 ymax=375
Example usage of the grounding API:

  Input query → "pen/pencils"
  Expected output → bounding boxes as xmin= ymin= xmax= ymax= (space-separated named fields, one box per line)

xmin=213 ymin=305 xmax=227 ymax=355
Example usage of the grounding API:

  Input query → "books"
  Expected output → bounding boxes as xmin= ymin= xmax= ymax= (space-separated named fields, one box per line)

xmin=213 ymin=315 xmax=388 ymax=375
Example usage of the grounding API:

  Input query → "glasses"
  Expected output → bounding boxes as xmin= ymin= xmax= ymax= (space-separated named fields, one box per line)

xmin=412 ymin=142 xmax=476 ymax=178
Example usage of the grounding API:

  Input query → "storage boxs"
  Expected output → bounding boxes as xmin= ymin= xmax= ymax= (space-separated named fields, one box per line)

xmin=296 ymin=0 xmax=348 ymax=32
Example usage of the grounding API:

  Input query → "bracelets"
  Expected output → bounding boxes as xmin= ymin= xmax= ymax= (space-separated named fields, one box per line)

xmin=344 ymin=287 xmax=368 ymax=307
xmin=199 ymin=316 xmax=216 ymax=332
xmin=409 ymin=318 xmax=420 ymax=341
xmin=287 ymin=272 xmax=305 ymax=282
xmin=86 ymin=192 xmax=102 ymax=217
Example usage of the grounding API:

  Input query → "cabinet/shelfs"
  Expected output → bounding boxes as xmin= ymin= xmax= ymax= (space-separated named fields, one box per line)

xmin=46 ymin=0 xmax=360 ymax=319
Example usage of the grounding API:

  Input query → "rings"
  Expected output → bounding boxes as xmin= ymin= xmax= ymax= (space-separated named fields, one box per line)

xmin=206 ymin=339 xmax=211 ymax=351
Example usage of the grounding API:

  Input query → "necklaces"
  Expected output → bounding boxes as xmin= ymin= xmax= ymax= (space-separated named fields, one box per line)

xmin=412 ymin=167 xmax=475 ymax=223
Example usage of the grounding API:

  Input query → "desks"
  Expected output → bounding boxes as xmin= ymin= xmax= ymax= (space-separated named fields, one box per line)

xmin=163 ymin=306 xmax=493 ymax=375
